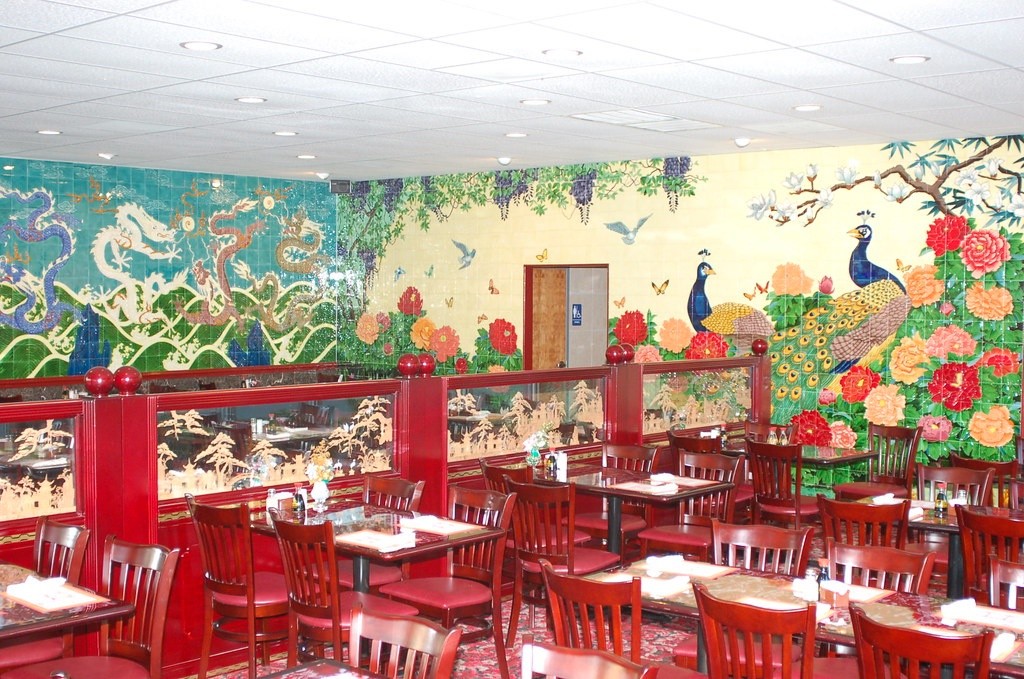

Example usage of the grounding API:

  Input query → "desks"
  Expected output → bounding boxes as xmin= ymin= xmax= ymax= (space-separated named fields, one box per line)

xmin=532 ymin=462 xmax=736 ymax=507
xmin=581 ymin=555 xmax=1024 ymax=676
xmin=720 ymin=422 xmax=879 ymax=465
xmin=0 ymin=579 xmax=136 ymax=642
xmin=250 ymin=500 xmax=505 ymax=565
xmin=448 ymin=414 xmax=505 ymax=431
xmin=242 ymin=420 xmax=339 ymax=449
xmin=852 ymin=495 xmax=1024 ymax=600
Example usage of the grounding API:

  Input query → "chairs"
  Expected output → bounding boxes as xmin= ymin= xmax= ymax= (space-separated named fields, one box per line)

xmin=0 ymin=373 xmax=1024 ymax=679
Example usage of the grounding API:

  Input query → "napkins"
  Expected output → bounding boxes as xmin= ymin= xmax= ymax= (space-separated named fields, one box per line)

xmin=474 ymin=409 xmax=491 ymax=420
xmin=643 ymin=554 xmax=665 ymax=578
xmin=250 ymin=417 xmax=271 ymax=429
xmin=252 ymin=426 xmax=290 ymax=441
xmin=22 ymin=459 xmax=71 ymax=468
xmin=942 ymin=598 xmax=977 ymax=626
xmin=281 ymin=425 xmax=312 ymax=434
xmin=379 ymin=531 xmax=416 ymax=552
xmin=40 ymin=577 xmax=66 ymax=592
xmin=652 ymin=484 xmax=678 ymax=496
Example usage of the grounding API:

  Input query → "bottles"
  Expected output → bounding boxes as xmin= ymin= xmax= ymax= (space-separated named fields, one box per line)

xmin=934 ymin=484 xmax=948 ymax=518
xmin=266 ymin=488 xmax=278 ymax=526
xmin=544 ymin=450 xmax=569 ymax=483
xmin=291 ymin=482 xmax=306 ymax=513
xmin=766 ymin=426 xmax=788 ymax=445
xmin=714 ymin=427 xmax=729 ymax=448
